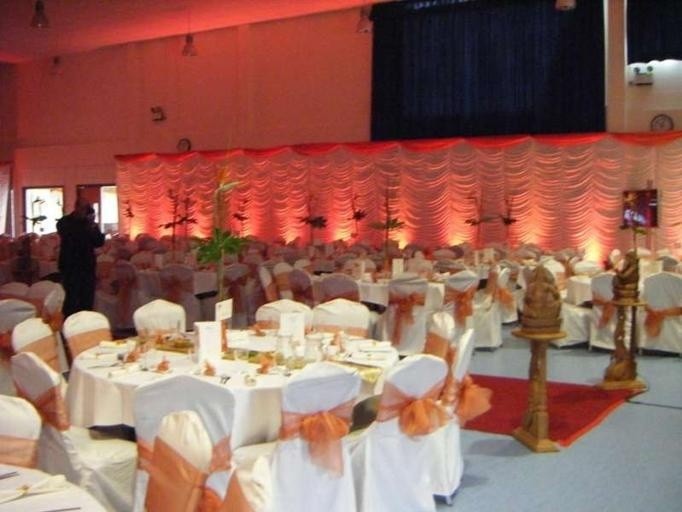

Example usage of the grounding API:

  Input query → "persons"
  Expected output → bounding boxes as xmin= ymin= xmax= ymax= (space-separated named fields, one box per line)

xmin=56 ymin=197 xmax=106 ymax=316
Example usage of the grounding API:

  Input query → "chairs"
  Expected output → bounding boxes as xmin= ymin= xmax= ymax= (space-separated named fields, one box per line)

xmin=143 ymin=409 xmax=213 ymax=512
xmin=432 ymin=327 xmax=475 ymax=506
xmin=230 ymin=358 xmax=363 ymax=510
xmin=0 ymin=232 xmax=682 ymax=360
xmin=8 ymin=351 xmax=138 ymax=511
xmin=135 ymin=373 xmax=238 ymax=512
xmin=11 ymin=317 xmax=58 ymax=380
xmin=1 ymin=394 xmax=44 ymax=467
xmin=347 ymin=353 xmax=450 ymax=511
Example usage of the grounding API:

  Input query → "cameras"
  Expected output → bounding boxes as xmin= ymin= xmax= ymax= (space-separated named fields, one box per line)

xmin=86 ymin=208 xmax=94 ymax=215
